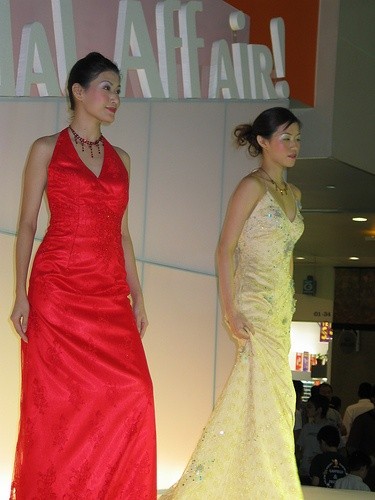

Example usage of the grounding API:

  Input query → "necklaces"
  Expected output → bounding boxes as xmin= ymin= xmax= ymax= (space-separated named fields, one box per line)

xmin=69 ymin=125 xmax=105 ymax=158
xmin=258 ymin=167 xmax=289 ymax=196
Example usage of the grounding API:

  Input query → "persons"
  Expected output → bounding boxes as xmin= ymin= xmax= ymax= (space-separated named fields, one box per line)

xmin=158 ymin=108 xmax=304 ymax=500
xmin=10 ymin=50 xmax=157 ymax=500
xmin=292 ymin=378 xmax=375 ymax=492
